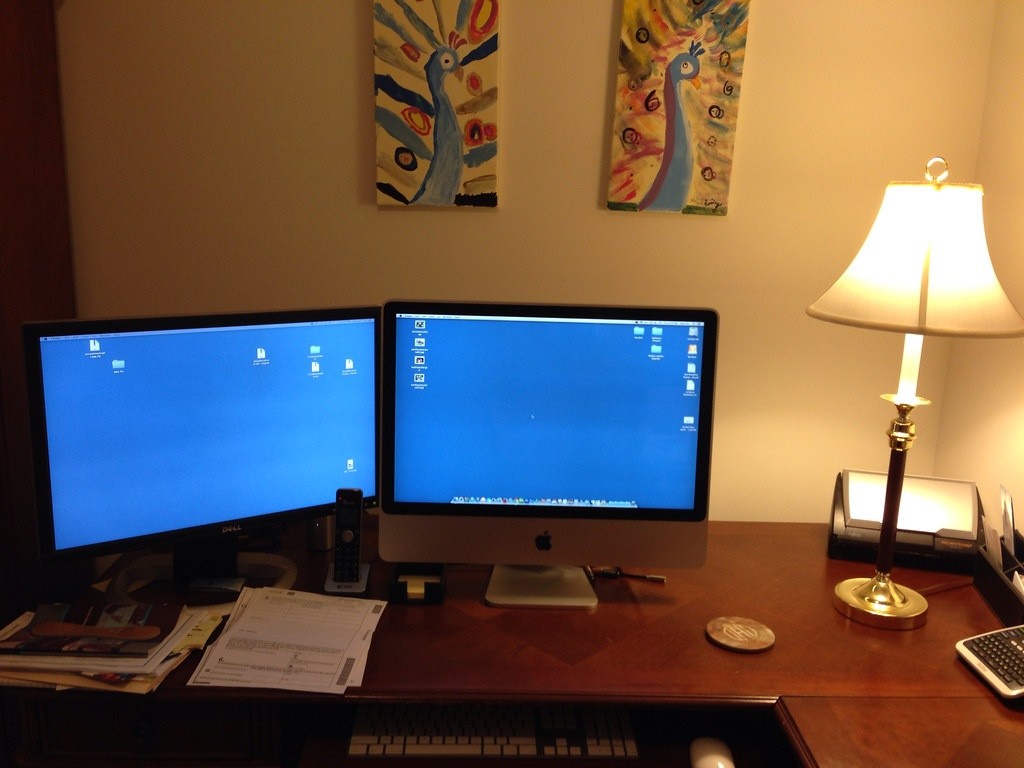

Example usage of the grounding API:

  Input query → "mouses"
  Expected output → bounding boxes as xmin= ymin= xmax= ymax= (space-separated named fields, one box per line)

xmin=689 ymin=736 xmax=736 ymax=768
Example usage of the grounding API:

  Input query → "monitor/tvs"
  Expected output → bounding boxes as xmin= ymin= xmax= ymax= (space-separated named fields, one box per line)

xmin=19 ymin=305 xmax=378 ymax=607
xmin=379 ymin=297 xmax=720 ymax=610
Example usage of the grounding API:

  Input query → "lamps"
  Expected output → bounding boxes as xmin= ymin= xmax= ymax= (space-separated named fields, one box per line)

xmin=806 ymin=157 xmax=1024 ymax=630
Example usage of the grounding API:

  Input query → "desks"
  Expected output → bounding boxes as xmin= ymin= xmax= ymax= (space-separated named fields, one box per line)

xmin=0 ymin=515 xmax=1024 ymax=768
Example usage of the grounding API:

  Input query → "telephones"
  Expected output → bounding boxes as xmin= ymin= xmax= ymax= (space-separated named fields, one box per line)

xmin=325 ymin=487 xmax=371 ymax=594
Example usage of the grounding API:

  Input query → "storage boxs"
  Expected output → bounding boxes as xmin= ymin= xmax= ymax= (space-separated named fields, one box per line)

xmin=973 ymin=529 xmax=1024 ymax=628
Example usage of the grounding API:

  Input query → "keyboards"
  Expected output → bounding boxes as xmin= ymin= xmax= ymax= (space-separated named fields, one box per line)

xmin=348 ymin=703 xmax=642 ymax=768
